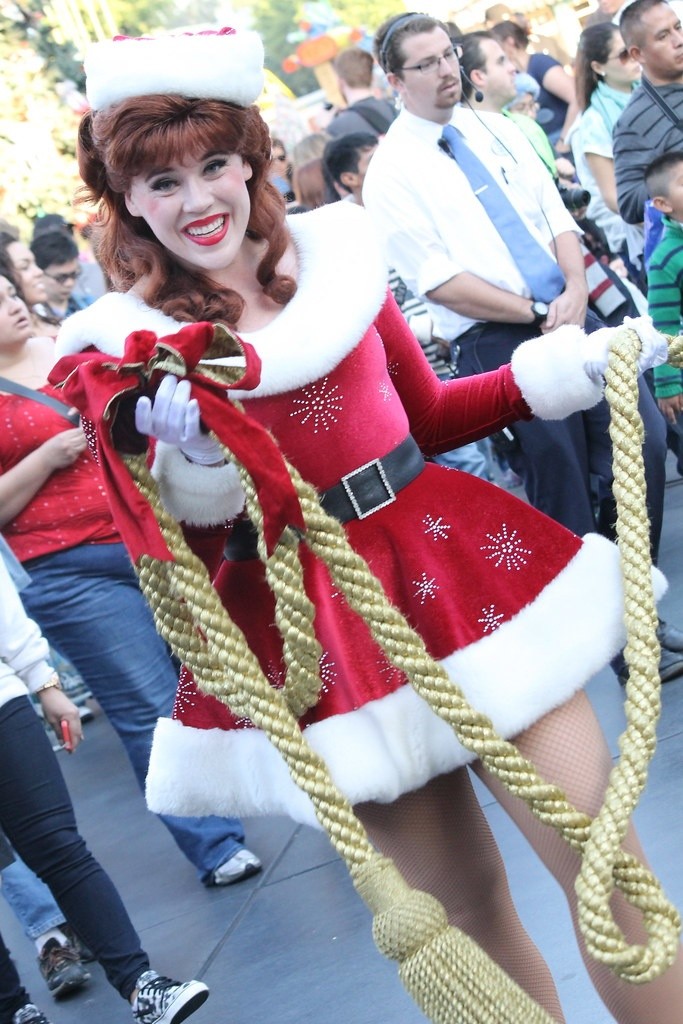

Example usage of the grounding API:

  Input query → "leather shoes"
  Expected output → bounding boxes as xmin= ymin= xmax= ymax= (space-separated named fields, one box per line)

xmin=611 ymin=615 xmax=683 ymax=690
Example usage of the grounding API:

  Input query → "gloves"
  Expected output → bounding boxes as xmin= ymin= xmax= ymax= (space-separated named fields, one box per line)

xmin=575 ymin=315 xmax=668 ymax=380
xmin=135 ymin=375 xmax=226 ymax=464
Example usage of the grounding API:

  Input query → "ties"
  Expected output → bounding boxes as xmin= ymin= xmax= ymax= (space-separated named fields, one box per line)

xmin=443 ymin=125 xmax=566 ymax=302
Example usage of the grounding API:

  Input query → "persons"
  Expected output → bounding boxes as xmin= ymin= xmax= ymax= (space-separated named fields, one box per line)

xmin=47 ymin=26 xmax=682 ymax=1022
xmin=1 ymin=0 xmax=683 ymax=1024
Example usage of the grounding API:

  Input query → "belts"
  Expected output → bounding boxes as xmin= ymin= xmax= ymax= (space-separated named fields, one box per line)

xmin=456 ymin=322 xmax=499 ymax=345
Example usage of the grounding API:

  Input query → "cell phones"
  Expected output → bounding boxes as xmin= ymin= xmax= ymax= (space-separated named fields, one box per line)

xmin=60 ymin=720 xmax=73 ymax=751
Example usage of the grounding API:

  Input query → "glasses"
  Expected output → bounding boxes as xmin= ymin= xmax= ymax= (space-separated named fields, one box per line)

xmin=398 ymin=42 xmax=464 ymax=74
xmin=607 ymin=49 xmax=630 ymax=65
xmin=272 ymin=155 xmax=286 ymax=161
xmin=43 ymin=263 xmax=83 ymax=283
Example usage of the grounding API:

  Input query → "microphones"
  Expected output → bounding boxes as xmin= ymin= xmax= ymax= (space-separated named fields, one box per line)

xmin=474 ymin=91 xmax=484 ymax=102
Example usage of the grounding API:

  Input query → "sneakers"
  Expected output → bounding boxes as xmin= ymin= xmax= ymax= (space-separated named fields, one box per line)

xmin=132 ymin=969 xmax=210 ymax=1024
xmin=13 ymin=1004 xmax=53 ymax=1024
xmin=36 ymin=922 xmax=97 ymax=996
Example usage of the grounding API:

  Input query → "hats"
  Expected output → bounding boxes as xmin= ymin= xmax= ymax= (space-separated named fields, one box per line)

xmin=82 ymin=22 xmax=265 ymax=109
xmin=482 ymin=3 xmax=513 ymax=24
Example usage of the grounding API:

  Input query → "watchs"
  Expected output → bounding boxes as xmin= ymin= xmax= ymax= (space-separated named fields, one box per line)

xmin=35 ymin=671 xmax=61 ymax=696
xmin=531 ymin=301 xmax=549 ymax=328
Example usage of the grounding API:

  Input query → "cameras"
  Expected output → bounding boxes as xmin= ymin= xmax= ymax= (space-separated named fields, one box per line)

xmin=553 ymin=175 xmax=591 ymax=211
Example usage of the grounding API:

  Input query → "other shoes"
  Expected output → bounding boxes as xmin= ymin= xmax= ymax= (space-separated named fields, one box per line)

xmin=213 ymin=847 xmax=263 ymax=885
xmin=79 ymin=706 xmax=93 ymax=722
